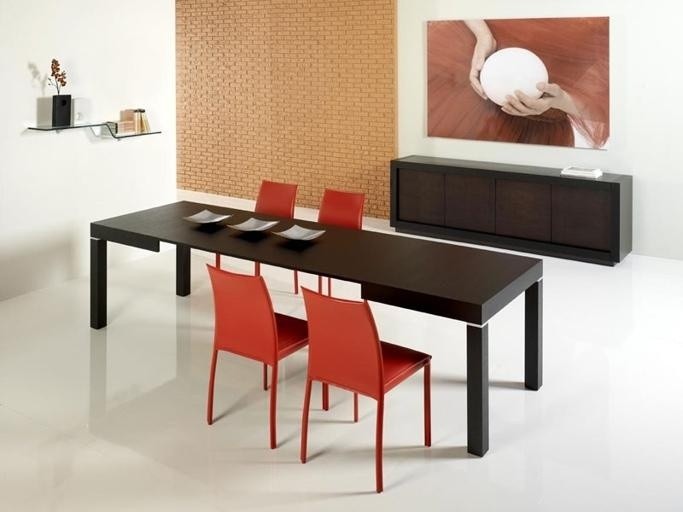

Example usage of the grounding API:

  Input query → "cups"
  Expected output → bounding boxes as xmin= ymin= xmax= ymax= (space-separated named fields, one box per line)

xmin=100 ymin=122 xmax=116 ymax=136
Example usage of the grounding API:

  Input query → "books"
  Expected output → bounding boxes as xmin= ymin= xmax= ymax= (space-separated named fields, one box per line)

xmin=100 ymin=109 xmax=151 ymax=137
xmin=561 ymin=166 xmax=602 ymax=178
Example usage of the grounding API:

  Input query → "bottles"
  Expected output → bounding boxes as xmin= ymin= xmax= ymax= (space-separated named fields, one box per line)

xmin=132 ymin=109 xmax=149 ymax=134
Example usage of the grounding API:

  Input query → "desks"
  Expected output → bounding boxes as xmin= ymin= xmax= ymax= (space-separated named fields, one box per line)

xmin=88 ymin=200 xmax=545 ymax=456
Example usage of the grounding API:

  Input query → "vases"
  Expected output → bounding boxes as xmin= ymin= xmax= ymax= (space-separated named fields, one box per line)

xmin=53 ymin=94 xmax=71 ymax=129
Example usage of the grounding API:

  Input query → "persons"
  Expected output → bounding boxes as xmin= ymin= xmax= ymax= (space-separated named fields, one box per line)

xmin=427 ymin=18 xmax=610 ymax=150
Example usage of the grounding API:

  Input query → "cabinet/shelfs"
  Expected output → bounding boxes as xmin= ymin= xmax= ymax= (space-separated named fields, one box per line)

xmin=388 ymin=154 xmax=633 ymax=269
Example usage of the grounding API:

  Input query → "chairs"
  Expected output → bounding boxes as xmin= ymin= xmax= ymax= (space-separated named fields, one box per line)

xmin=293 ymin=187 xmax=366 ymax=298
xmin=212 ymin=179 xmax=298 ymax=296
xmin=204 ymin=264 xmax=328 ymax=448
xmin=298 ymin=284 xmax=433 ymax=493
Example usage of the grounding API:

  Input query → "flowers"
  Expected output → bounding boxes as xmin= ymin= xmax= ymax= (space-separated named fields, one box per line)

xmin=48 ymin=57 xmax=67 ymax=95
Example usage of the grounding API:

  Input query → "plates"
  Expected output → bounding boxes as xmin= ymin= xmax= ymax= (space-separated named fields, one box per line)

xmin=225 ymin=217 xmax=281 ymax=233
xmin=269 ymin=224 xmax=327 ymax=243
xmin=180 ymin=208 xmax=233 ymax=225
